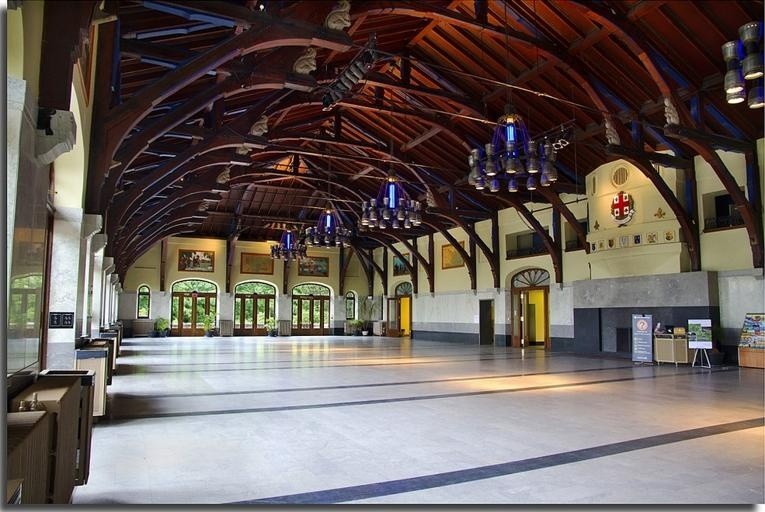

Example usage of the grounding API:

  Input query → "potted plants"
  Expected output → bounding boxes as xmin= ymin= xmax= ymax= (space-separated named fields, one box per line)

xmin=265 ymin=318 xmax=277 ymax=337
xmin=360 ymin=296 xmax=380 ymax=335
xmin=203 ymin=315 xmax=214 ymax=337
xmin=155 ymin=318 xmax=169 ymax=337
xmin=348 ymin=319 xmax=363 ymax=336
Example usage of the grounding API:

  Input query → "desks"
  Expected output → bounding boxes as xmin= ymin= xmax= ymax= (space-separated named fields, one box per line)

xmin=654 ymin=334 xmax=696 ymax=366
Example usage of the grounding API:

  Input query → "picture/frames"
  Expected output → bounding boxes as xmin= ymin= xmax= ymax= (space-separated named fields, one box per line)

xmin=240 ymin=252 xmax=273 ymax=275
xmin=298 ymin=256 xmax=329 ymax=278
xmin=393 ymin=253 xmax=410 ymax=276
xmin=178 ymin=249 xmax=214 ymax=273
xmin=442 ymin=241 xmax=464 ymax=269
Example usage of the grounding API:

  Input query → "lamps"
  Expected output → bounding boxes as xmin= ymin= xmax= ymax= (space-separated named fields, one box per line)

xmin=721 ymin=19 xmax=764 ymax=109
xmin=361 ymin=62 xmax=422 ymax=229
xmin=468 ymin=1 xmax=559 ymax=192
xmin=321 ymin=32 xmax=377 ymax=112
xmin=271 ymin=183 xmax=306 ymax=261
xmin=304 ymin=148 xmax=352 ymax=248
xmin=531 ymin=119 xmax=576 ymax=154
